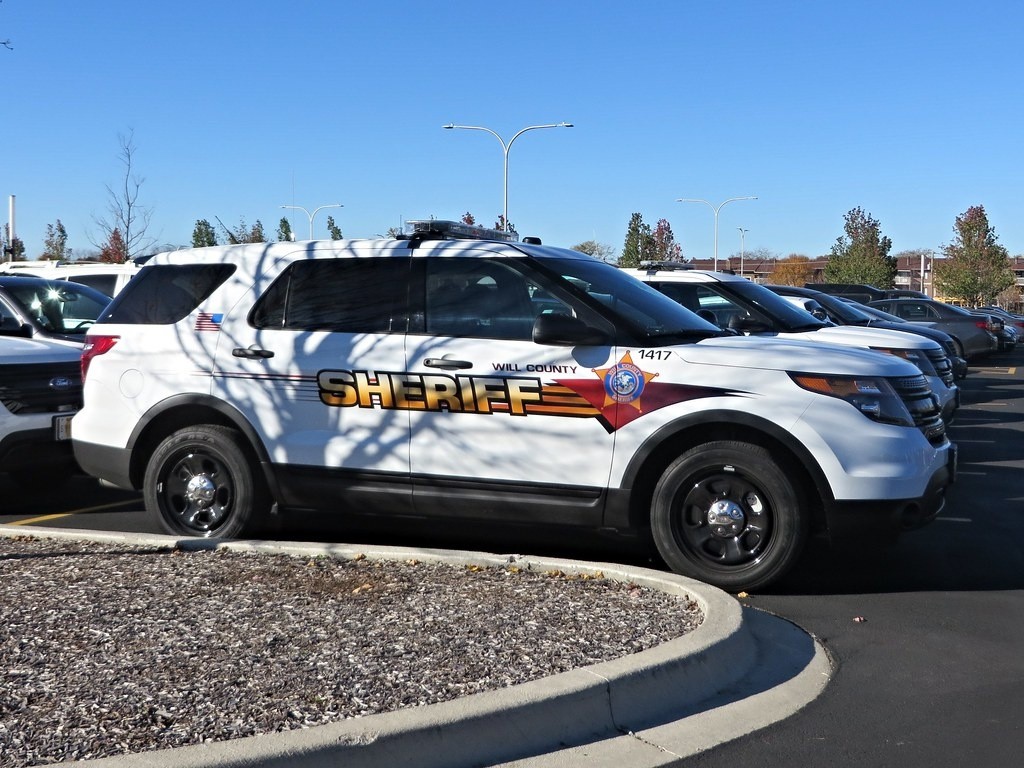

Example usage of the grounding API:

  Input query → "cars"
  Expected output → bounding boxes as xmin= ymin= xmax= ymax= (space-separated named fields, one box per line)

xmin=693 ymin=283 xmax=1024 ymax=381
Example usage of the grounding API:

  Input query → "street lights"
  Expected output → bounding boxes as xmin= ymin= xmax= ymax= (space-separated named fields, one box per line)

xmin=443 ymin=122 xmax=575 ymax=233
xmin=735 ymin=227 xmax=749 ymax=277
xmin=675 ymin=196 xmax=759 ymax=275
xmin=278 ymin=204 xmax=345 ymax=241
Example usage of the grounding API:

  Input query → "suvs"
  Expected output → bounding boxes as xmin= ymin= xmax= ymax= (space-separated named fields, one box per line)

xmin=611 ymin=259 xmax=962 ymax=429
xmin=69 ymin=238 xmax=959 ymax=595
xmin=0 ymin=257 xmax=155 ymax=471
